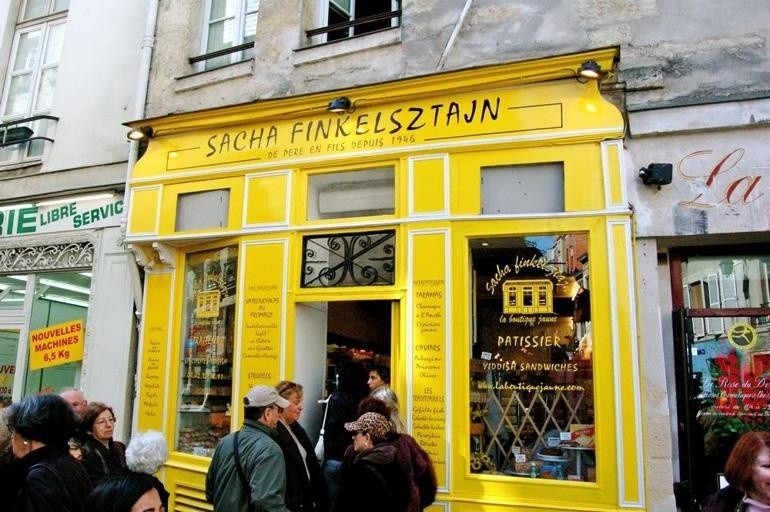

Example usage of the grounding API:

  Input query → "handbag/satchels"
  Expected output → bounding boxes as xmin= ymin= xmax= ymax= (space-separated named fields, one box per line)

xmin=313 ymin=428 xmax=325 ymax=463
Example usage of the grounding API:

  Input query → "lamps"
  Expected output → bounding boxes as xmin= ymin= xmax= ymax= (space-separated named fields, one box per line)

xmin=326 ymin=96 xmax=352 ymax=113
xmin=126 ymin=125 xmax=155 ymax=143
xmin=576 ymin=59 xmax=603 ymax=80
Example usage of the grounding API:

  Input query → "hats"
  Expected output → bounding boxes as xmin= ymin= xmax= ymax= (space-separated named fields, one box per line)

xmin=241 ymin=383 xmax=291 ymax=410
xmin=344 ymin=410 xmax=393 ymax=439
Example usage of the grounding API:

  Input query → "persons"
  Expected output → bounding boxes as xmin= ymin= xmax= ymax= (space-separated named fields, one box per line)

xmin=703 ymin=430 xmax=770 ymax=512
xmin=0 ymin=393 xmax=170 ymax=512
xmin=205 ymin=366 xmax=439 ymax=512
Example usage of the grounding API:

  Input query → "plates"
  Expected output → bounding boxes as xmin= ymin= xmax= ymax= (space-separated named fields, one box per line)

xmin=536 ymin=453 xmax=572 ymax=463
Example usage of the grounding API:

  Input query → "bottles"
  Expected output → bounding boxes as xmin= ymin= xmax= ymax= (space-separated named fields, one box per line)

xmin=186 ymin=339 xmax=197 ymax=359
